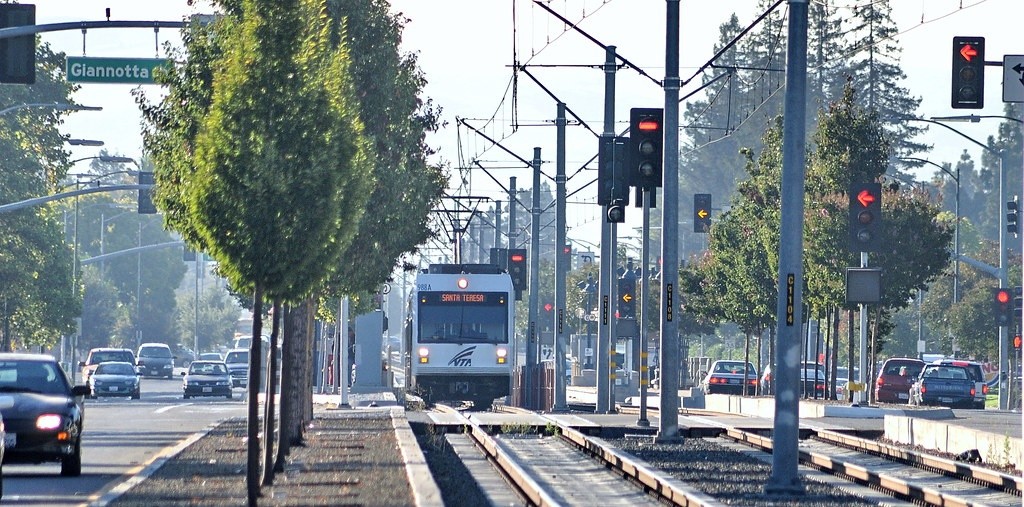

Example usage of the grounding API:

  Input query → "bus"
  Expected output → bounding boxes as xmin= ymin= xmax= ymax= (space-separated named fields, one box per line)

xmin=405 ymin=263 xmax=515 ymax=411
xmin=235 ymin=319 xmax=253 ymax=339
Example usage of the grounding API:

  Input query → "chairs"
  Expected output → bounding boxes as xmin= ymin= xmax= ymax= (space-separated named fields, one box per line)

xmin=951 ymin=373 xmax=963 ymax=378
xmin=890 ymin=370 xmax=898 ymax=374
xmin=95 ymin=357 xmax=102 ymax=361
xmin=195 ymin=370 xmax=203 ymax=373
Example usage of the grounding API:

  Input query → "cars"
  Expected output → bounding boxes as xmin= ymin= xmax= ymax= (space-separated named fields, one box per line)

xmin=702 ymin=357 xmax=987 ymax=410
xmin=172 ymin=336 xmax=268 ymax=400
xmin=0 ymin=353 xmax=91 ymax=475
xmin=78 ymin=348 xmax=144 ymax=400
xmin=382 ymin=336 xmax=400 ymax=352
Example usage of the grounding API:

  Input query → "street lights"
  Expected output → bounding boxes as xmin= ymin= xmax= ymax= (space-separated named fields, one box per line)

xmin=576 ymin=257 xmax=641 ymax=369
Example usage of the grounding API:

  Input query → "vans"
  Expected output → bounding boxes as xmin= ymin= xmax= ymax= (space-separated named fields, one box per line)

xmin=135 ymin=343 xmax=177 ymax=379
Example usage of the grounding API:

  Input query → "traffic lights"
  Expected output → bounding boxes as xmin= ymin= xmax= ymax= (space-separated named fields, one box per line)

xmin=844 ymin=267 xmax=881 ymax=305
xmin=508 ymin=249 xmax=526 ymax=290
xmin=996 ymin=286 xmax=1022 ymax=349
xmin=565 ymin=245 xmax=571 ymax=270
xmin=694 ymin=194 xmax=711 ymax=233
xmin=618 ymin=277 xmax=636 ymax=317
xmin=543 ymin=299 xmax=553 ymax=317
xmin=1006 ymin=201 xmax=1018 ymax=233
xmin=846 ymin=183 xmax=883 ymax=251
xmin=951 ymin=36 xmax=985 ymax=109
xmin=629 ymin=108 xmax=663 ymax=188
